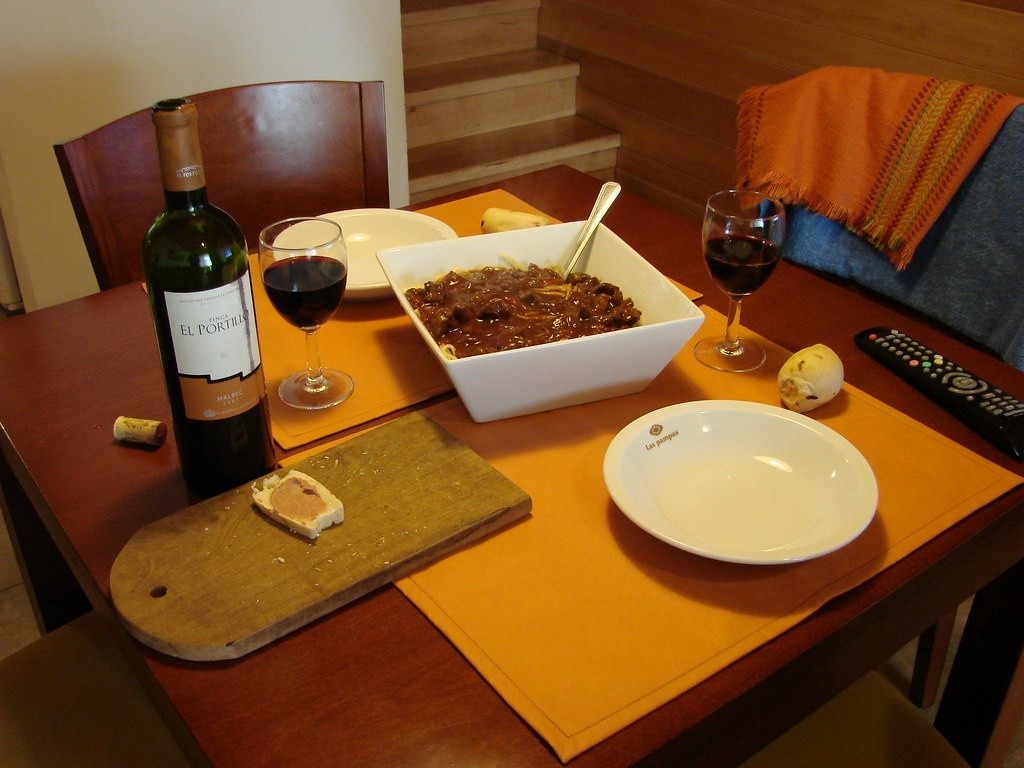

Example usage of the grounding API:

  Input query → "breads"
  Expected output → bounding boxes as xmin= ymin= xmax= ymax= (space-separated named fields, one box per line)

xmin=479 ymin=206 xmax=551 ymax=233
xmin=777 ymin=343 xmax=843 ymax=413
xmin=251 ymin=469 xmax=345 ymax=540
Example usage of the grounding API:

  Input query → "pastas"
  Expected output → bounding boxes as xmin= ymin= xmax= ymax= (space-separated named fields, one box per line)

xmin=413 ymin=253 xmax=577 ymax=360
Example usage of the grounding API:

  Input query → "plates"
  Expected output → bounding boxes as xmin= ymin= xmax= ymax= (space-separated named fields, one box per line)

xmin=273 ymin=208 xmax=457 ymax=298
xmin=602 ymin=399 xmax=879 ymax=564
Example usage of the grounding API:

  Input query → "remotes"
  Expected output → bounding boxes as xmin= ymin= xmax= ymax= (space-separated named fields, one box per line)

xmin=855 ymin=325 xmax=1023 ymax=461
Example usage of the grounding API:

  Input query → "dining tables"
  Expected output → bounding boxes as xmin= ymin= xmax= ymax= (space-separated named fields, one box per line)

xmin=0 ymin=164 xmax=1024 ymax=768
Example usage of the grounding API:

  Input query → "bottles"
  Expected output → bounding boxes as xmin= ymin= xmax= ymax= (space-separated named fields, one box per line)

xmin=141 ymin=99 xmax=279 ymax=506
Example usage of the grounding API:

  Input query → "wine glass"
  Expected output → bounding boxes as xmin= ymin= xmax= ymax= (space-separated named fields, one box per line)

xmin=694 ymin=191 xmax=786 ymax=374
xmin=257 ymin=217 xmax=355 ymax=409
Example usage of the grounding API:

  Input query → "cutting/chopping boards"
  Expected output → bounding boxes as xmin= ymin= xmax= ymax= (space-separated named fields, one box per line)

xmin=109 ymin=409 xmax=532 ymax=661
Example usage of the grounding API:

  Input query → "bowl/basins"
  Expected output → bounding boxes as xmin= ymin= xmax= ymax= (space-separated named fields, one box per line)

xmin=374 ymin=221 xmax=707 ymax=422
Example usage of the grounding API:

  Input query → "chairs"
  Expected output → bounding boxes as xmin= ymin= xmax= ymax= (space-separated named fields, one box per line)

xmin=52 ymin=81 xmax=393 ymax=291
xmin=0 ymin=609 xmax=193 ymax=766
xmin=748 ymin=65 xmax=1022 ymax=707
xmin=744 ymin=671 xmax=972 ymax=766
